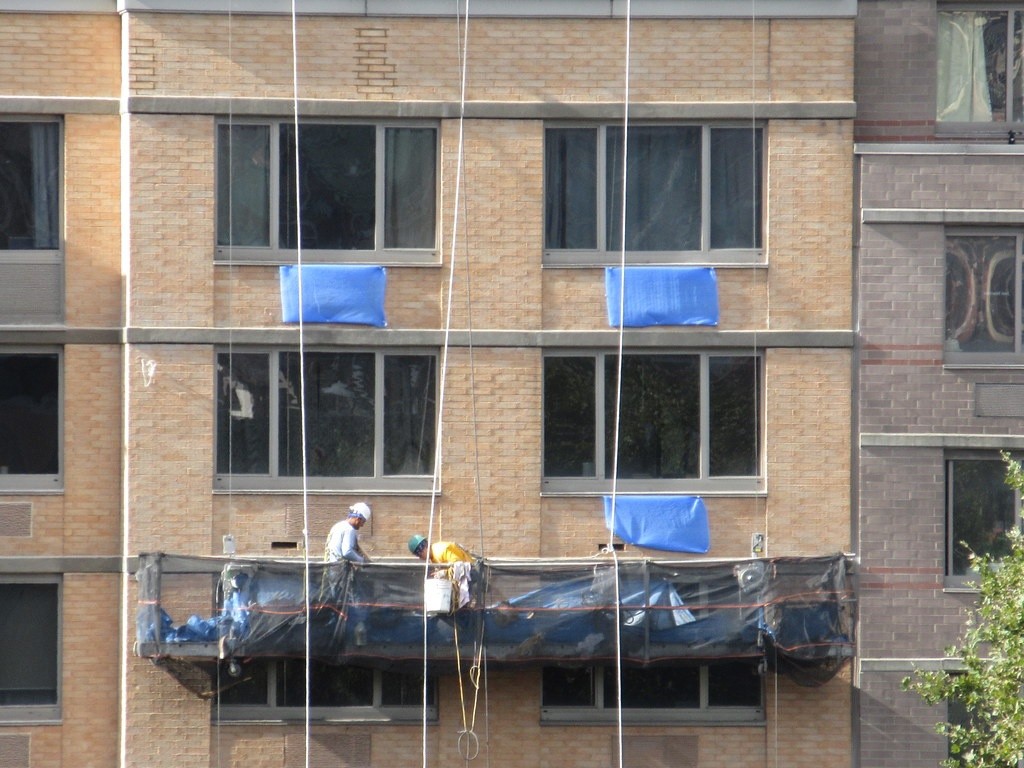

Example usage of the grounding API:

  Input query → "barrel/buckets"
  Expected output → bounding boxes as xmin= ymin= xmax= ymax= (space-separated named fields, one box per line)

xmin=424 ymin=579 xmax=452 ymax=613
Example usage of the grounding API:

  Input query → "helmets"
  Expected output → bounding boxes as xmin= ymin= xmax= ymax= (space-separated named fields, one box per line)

xmin=408 ymin=535 xmax=425 ymax=554
xmin=349 ymin=502 xmax=371 ymax=522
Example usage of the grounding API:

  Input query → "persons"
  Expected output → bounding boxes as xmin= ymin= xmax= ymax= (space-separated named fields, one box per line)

xmin=326 ymin=502 xmax=374 ymax=604
xmin=409 ymin=535 xmax=477 ymax=608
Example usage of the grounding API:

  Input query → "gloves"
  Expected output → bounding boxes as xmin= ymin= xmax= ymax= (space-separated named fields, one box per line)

xmin=362 ymin=559 xmax=372 ymax=569
xmin=430 ymin=570 xmax=445 ymax=579
